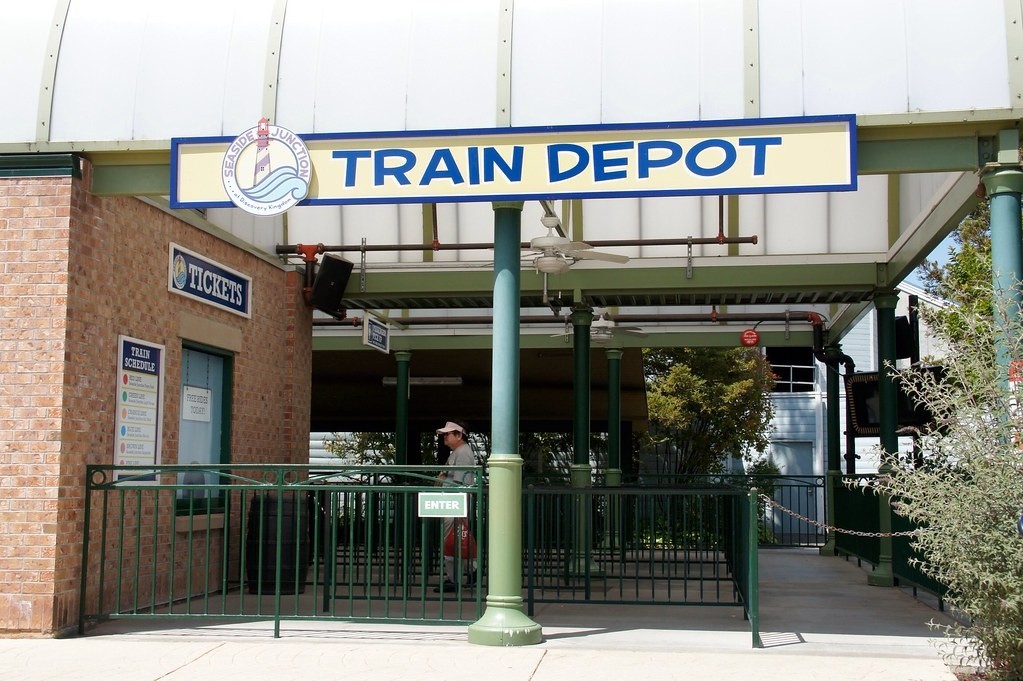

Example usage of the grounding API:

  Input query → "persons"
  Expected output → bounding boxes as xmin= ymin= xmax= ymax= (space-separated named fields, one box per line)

xmin=433 ymin=417 xmax=477 ymax=592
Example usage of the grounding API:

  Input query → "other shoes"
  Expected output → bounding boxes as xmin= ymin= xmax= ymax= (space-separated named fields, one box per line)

xmin=433 ymin=579 xmax=458 ymax=593
xmin=464 ymin=569 xmax=485 ymax=588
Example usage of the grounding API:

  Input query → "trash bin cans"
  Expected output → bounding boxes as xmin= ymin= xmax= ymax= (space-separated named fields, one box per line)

xmin=246 ymin=499 xmax=308 ymax=595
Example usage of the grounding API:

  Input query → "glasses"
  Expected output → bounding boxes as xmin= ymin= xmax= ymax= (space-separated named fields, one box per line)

xmin=443 ymin=432 xmax=457 ymax=436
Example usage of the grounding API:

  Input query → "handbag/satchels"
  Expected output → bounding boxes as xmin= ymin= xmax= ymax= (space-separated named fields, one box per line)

xmin=445 ymin=518 xmax=477 ymax=558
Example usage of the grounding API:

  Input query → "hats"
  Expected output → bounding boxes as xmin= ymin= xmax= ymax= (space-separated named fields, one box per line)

xmin=436 ymin=421 xmax=468 ymax=437
